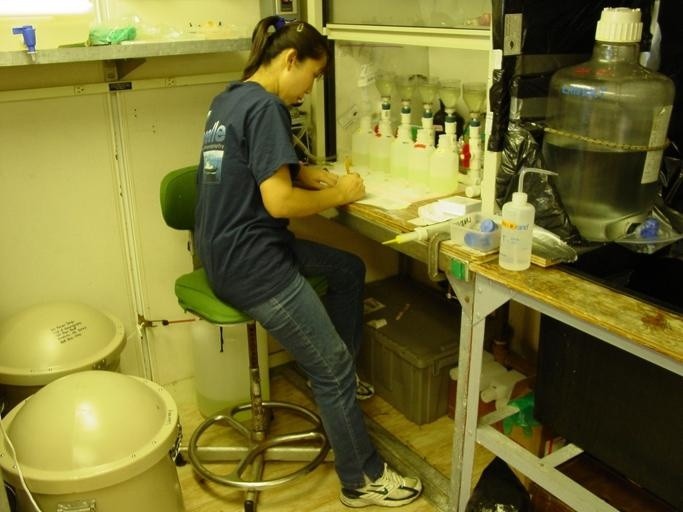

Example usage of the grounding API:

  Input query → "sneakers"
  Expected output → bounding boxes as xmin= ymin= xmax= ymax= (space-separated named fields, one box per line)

xmin=306 ymin=372 xmax=375 ymax=400
xmin=339 ymin=462 xmax=422 ymax=508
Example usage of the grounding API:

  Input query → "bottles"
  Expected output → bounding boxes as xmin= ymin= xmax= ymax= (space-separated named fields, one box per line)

xmin=349 ymin=73 xmax=487 ymax=196
xmin=498 ymin=168 xmax=560 ymax=272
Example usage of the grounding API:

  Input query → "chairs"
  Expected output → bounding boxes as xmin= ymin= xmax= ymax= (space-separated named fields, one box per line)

xmin=154 ymin=162 xmax=337 ymax=512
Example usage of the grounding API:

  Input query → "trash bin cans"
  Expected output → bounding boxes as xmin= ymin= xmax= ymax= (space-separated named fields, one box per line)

xmin=0 ymin=298 xmax=183 ymax=511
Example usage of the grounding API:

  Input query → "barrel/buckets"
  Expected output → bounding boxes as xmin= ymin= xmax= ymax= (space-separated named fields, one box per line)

xmin=190 ymin=316 xmax=271 ymax=425
xmin=542 ymin=6 xmax=676 ymax=242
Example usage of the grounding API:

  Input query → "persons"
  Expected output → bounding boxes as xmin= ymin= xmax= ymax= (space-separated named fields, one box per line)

xmin=194 ymin=16 xmax=423 ymax=507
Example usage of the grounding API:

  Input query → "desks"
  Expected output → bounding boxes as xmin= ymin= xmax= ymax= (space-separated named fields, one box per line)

xmin=457 ymin=198 xmax=683 ymax=511
xmin=313 ymin=160 xmax=515 ymax=511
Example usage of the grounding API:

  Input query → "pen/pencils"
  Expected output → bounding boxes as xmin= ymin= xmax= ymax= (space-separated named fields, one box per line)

xmin=396 ymin=303 xmax=410 ymax=321
xmin=344 ymin=156 xmax=350 ymax=173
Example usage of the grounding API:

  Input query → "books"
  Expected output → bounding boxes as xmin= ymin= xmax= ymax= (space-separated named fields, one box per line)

xmin=316 ymin=161 xmax=416 ymax=212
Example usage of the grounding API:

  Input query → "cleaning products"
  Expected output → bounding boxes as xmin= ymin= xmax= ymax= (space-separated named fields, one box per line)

xmin=351 ymin=116 xmax=459 ymax=195
